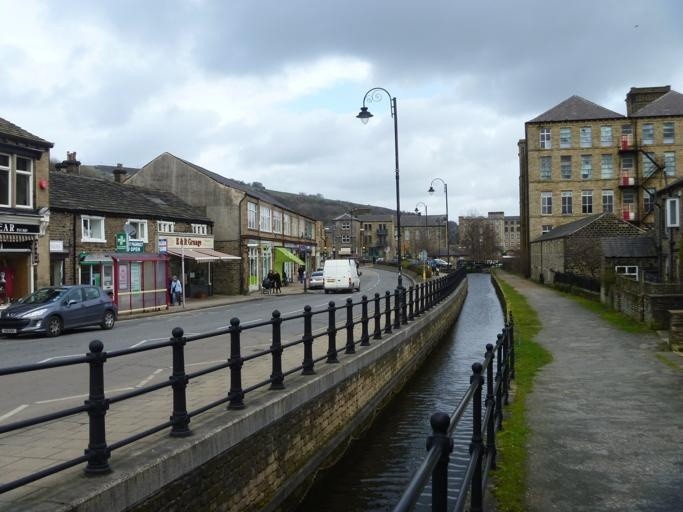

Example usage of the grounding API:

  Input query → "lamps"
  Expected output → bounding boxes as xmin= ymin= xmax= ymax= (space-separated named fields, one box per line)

xmin=73 ymin=253 xmax=86 ymax=261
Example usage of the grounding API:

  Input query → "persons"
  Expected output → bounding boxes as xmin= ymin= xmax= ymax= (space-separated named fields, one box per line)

xmin=297 ymin=265 xmax=304 ymax=283
xmin=273 ymin=271 xmax=281 ymax=293
xmin=261 ymin=276 xmax=273 ymax=290
xmin=169 ymin=275 xmax=182 ymax=306
xmin=267 ymin=269 xmax=274 ymax=280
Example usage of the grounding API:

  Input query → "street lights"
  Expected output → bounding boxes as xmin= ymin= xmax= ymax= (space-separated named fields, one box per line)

xmin=332 ymin=246 xmax=336 ymax=259
xmin=429 ymin=178 xmax=450 ymax=275
xmin=356 ymin=88 xmax=406 ymax=324
xmin=415 ymin=200 xmax=427 ymax=232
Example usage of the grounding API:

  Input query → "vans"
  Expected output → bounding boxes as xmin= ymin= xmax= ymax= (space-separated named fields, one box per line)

xmin=323 ymin=259 xmax=361 ymax=293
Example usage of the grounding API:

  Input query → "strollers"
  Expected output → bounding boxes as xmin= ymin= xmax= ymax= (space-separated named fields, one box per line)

xmin=262 ymin=277 xmax=273 ymax=295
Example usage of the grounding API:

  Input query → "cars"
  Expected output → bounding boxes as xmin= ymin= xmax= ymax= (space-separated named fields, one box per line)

xmin=309 ymin=270 xmax=324 ymax=289
xmin=0 ymin=285 xmax=117 ymax=337
xmin=426 ymin=259 xmax=452 ymax=270
